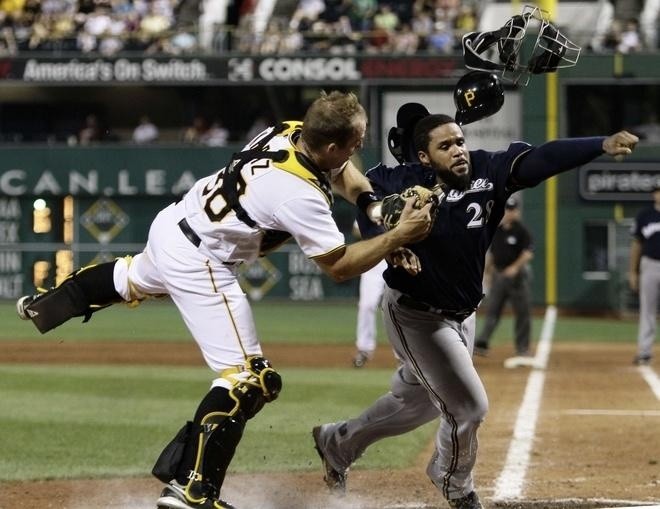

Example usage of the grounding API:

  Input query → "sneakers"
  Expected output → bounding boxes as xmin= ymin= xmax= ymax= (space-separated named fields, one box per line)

xmin=156 ymin=474 xmax=238 ymax=509
xmin=445 ymin=489 xmax=483 ymax=508
xmin=16 ymin=286 xmax=57 ymax=319
xmin=472 ymin=340 xmax=488 ymax=355
xmin=312 ymin=425 xmax=348 ymax=492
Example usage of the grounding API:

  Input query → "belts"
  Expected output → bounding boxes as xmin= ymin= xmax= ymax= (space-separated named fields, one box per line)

xmin=175 ymin=197 xmax=200 ymax=247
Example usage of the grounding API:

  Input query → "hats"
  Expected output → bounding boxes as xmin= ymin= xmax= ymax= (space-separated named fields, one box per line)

xmin=387 ymin=103 xmax=430 ymax=164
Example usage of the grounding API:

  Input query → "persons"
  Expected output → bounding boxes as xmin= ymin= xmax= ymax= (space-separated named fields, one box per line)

xmin=351 ymin=198 xmax=406 ymax=367
xmin=312 ymin=103 xmax=640 ymax=509
xmin=1 ymin=2 xmax=659 ymax=149
xmin=473 ymin=195 xmax=538 ymax=359
xmin=15 ymin=90 xmax=433 ymax=509
xmin=627 ymin=181 xmax=659 ymax=365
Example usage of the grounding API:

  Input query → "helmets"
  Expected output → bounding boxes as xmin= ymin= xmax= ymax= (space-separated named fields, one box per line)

xmin=452 ymin=69 xmax=506 ymax=126
